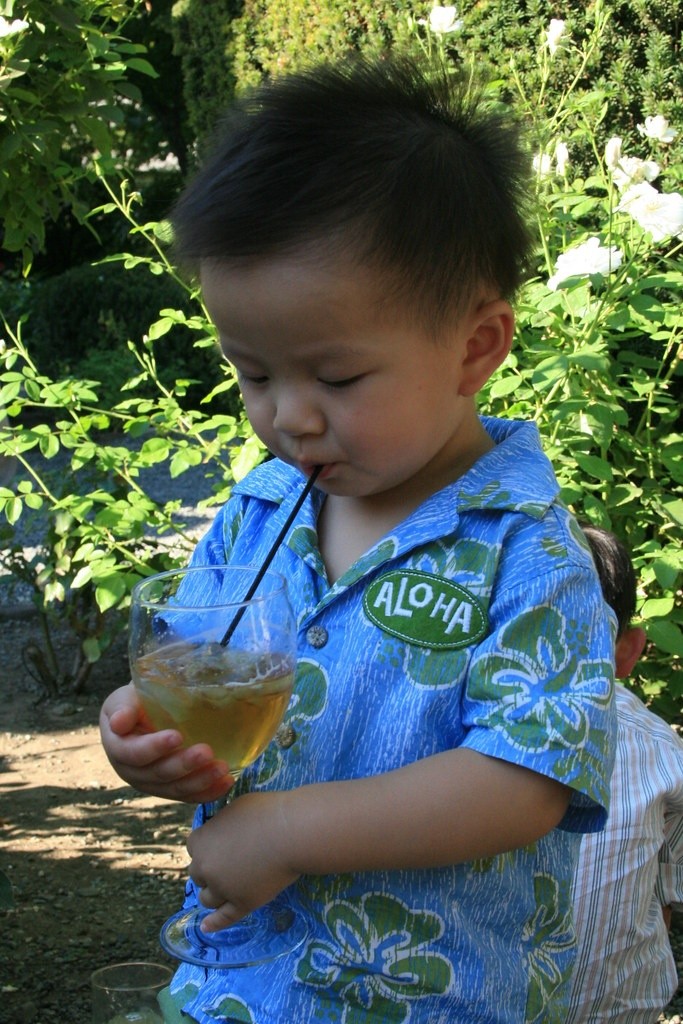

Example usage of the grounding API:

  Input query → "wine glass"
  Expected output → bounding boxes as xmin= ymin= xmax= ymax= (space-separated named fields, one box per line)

xmin=127 ymin=566 xmax=308 ymax=966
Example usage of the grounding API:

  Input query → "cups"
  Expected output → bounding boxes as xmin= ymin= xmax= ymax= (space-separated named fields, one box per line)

xmin=90 ymin=962 xmax=185 ymax=1024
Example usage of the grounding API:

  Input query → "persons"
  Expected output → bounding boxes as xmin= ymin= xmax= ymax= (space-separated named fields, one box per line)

xmin=100 ymin=57 xmax=617 ymax=1024
xmin=564 ymin=525 xmax=683 ymax=1024
